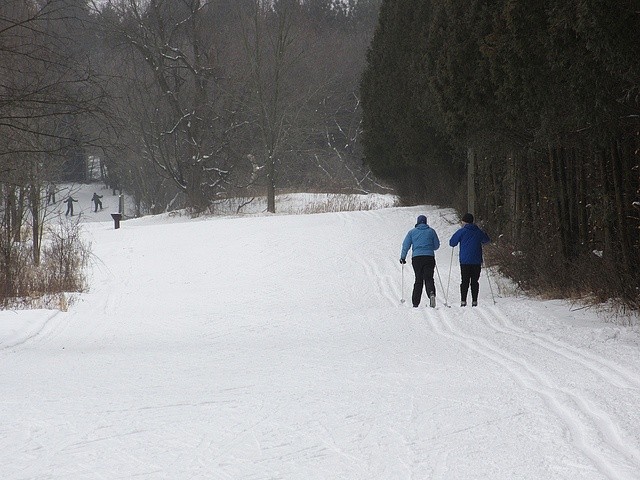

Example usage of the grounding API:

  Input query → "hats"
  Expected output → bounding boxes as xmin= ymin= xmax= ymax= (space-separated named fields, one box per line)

xmin=417 ymin=215 xmax=427 ymax=223
xmin=462 ymin=214 xmax=473 ymax=223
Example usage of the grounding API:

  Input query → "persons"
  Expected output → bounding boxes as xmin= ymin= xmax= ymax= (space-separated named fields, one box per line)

xmin=63 ymin=194 xmax=75 ymax=216
xmin=47 ymin=181 xmax=58 ymax=204
xmin=449 ymin=213 xmax=488 ymax=306
xmin=400 ymin=216 xmax=440 ymax=308
xmin=91 ymin=192 xmax=104 ymax=211
